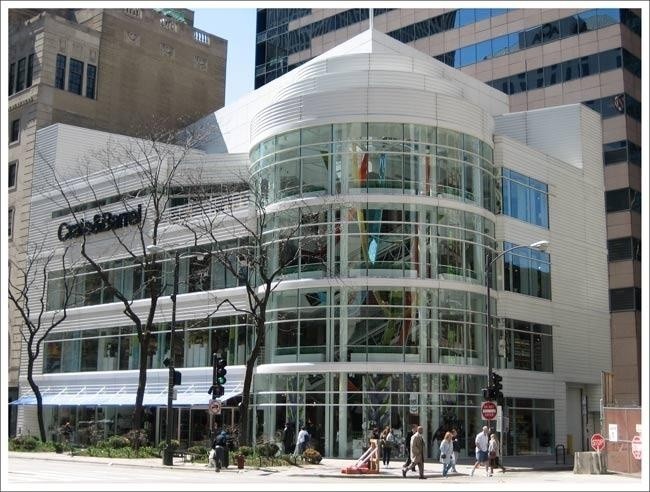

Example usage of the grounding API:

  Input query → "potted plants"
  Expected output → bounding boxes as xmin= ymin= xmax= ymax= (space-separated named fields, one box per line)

xmin=159 ymin=438 xmax=180 ymax=465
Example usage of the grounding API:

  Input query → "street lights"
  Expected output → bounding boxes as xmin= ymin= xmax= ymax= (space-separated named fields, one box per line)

xmin=147 ymin=245 xmax=205 ymax=444
xmin=485 ymin=240 xmax=550 ymax=379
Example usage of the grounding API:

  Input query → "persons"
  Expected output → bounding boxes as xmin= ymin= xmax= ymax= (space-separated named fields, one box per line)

xmin=385 ymin=429 xmax=394 ymax=447
xmin=282 ymin=422 xmax=294 ymax=454
xmin=295 ymin=426 xmax=310 ymax=452
xmin=402 ymin=425 xmax=427 ymax=479
xmin=485 ymin=426 xmax=496 ymax=450
xmin=63 ymin=421 xmax=74 ymax=443
xmin=381 ymin=425 xmax=394 ymax=465
xmin=404 ymin=423 xmax=417 ymax=471
xmin=447 ymin=426 xmax=461 ymax=473
xmin=487 ymin=433 xmax=507 ymax=474
xmin=467 ymin=425 xmax=490 ymax=477
xmin=437 ymin=430 xmax=455 ymax=477
xmin=207 ymin=429 xmax=231 ymax=472
xmin=432 ymin=424 xmax=448 ymax=461
xmin=369 ymin=427 xmax=380 ymax=443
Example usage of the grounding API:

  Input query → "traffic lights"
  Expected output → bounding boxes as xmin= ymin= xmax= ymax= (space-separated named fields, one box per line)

xmin=482 ymin=373 xmax=503 ymax=401
xmin=212 ymin=353 xmax=227 ymax=399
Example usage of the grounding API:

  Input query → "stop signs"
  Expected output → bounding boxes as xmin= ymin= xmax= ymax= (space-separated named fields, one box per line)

xmin=590 ymin=433 xmax=607 ymax=451
xmin=482 ymin=402 xmax=498 ymax=420
